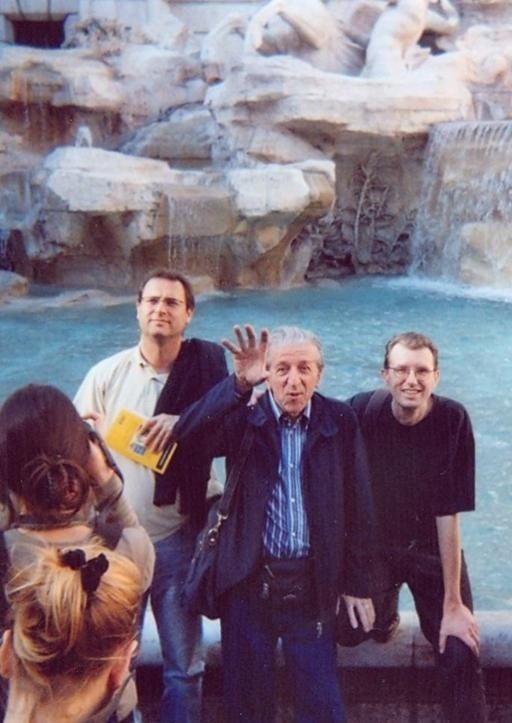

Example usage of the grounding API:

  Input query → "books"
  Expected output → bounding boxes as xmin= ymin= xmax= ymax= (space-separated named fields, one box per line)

xmin=103 ymin=408 xmax=178 ymax=476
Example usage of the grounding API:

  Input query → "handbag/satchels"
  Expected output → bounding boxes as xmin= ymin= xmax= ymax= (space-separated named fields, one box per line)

xmin=179 ymin=410 xmax=257 ymax=618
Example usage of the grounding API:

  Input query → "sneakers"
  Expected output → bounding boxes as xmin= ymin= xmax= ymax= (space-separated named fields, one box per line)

xmin=370 ymin=612 xmax=399 ymax=642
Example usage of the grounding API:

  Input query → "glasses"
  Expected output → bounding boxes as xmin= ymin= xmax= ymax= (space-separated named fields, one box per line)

xmin=141 ymin=295 xmax=185 ymax=306
xmin=387 ymin=365 xmax=438 ymax=378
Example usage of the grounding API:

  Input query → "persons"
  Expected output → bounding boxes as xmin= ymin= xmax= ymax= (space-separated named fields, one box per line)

xmin=72 ymin=270 xmax=229 ymax=723
xmin=0 ymin=537 xmax=143 ymax=723
xmin=170 ymin=324 xmax=375 ymax=723
xmin=336 ymin=332 xmax=488 ymax=723
xmin=1 ymin=384 xmax=155 ymax=723
